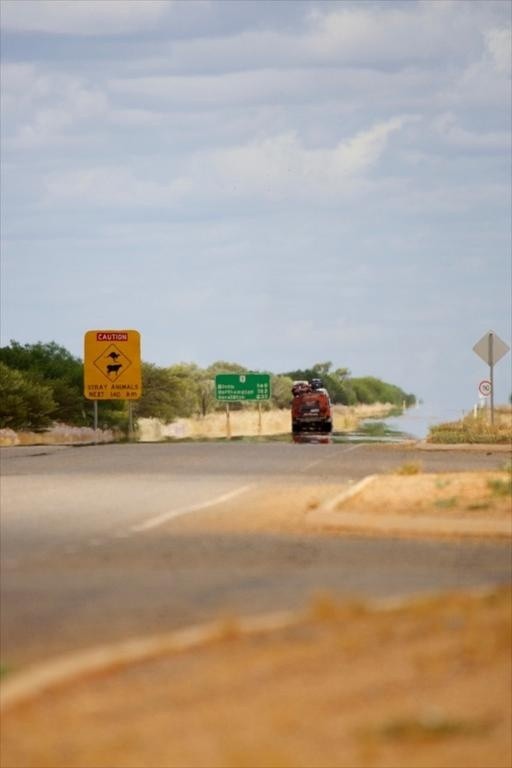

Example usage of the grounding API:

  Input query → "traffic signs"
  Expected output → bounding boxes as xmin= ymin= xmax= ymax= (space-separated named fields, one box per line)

xmin=216 ymin=374 xmax=270 ymax=400
xmin=478 ymin=380 xmax=494 ymax=397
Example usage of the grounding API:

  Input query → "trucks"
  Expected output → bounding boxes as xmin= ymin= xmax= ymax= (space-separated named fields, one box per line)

xmin=289 ymin=380 xmax=332 ymax=432
